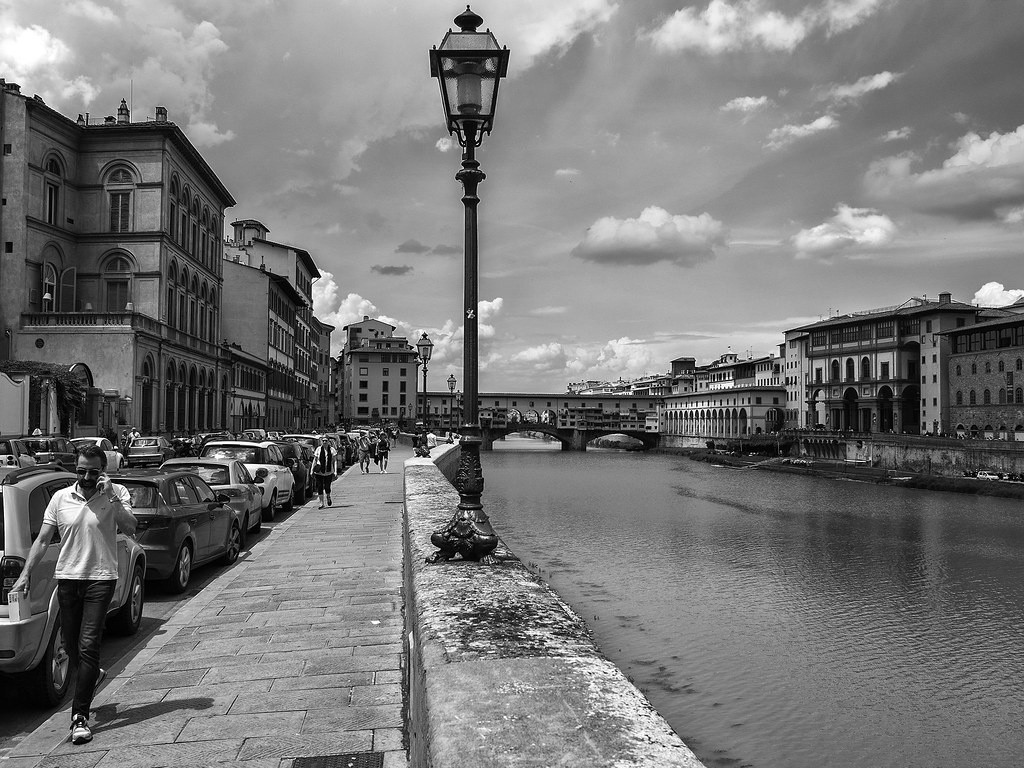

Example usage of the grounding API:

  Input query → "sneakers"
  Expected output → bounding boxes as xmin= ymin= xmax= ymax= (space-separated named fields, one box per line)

xmin=90 ymin=669 xmax=108 ymax=702
xmin=69 ymin=713 xmax=93 ymax=745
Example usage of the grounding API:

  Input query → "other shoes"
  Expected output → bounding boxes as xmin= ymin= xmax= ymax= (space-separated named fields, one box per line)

xmin=383 ymin=470 xmax=387 ymax=474
xmin=366 ymin=467 xmax=369 ymax=474
xmin=327 ymin=496 xmax=332 ymax=506
xmin=319 ymin=502 xmax=324 ymax=508
xmin=361 ymin=470 xmax=364 ymax=474
xmin=380 ymin=470 xmax=383 ymax=475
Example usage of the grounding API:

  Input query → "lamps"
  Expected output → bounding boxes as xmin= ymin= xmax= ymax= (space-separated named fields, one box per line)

xmin=37 ymin=293 xmax=52 ymax=303
xmin=124 ymin=302 xmax=133 ymax=311
xmin=85 ymin=303 xmax=92 ymax=312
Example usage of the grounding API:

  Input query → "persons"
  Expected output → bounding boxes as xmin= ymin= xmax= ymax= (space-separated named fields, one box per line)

xmin=426 ymin=429 xmax=437 ymax=449
xmin=283 ymin=426 xmax=304 ymax=434
xmin=375 ymin=434 xmax=390 ymax=475
xmin=31 ymin=428 xmax=43 ymax=438
xmin=310 ymin=437 xmax=338 ymax=509
xmin=121 ymin=427 xmax=141 ymax=459
xmin=357 ymin=432 xmax=372 ymax=475
xmin=412 ymin=431 xmax=422 ymax=457
xmin=311 ymin=427 xmax=317 ymax=434
xmin=185 ymin=433 xmax=203 ymax=449
xmin=391 ymin=427 xmax=397 ymax=448
xmin=223 ymin=427 xmax=236 ymax=440
xmin=9 ymin=445 xmax=138 ymax=745
xmin=148 ymin=428 xmax=153 ymax=436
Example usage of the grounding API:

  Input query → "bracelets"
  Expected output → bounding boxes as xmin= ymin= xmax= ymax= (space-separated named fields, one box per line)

xmin=109 ymin=498 xmax=120 ymax=503
xmin=108 ymin=493 xmax=117 ymax=502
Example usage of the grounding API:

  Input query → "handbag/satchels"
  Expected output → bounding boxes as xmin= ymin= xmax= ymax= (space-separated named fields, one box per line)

xmin=314 ymin=465 xmax=322 ymax=473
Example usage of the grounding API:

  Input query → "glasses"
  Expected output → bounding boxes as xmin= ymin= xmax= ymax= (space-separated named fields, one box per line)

xmin=76 ymin=467 xmax=103 ymax=475
xmin=322 ymin=440 xmax=327 ymax=442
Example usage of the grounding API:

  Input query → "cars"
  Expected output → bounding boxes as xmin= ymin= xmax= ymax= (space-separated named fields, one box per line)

xmin=18 ymin=437 xmax=80 ymax=474
xmin=197 ymin=438 xmax=297 ymax=522
xmin=0 ymin=438 xmax=39 ymax=494
xmin=976 ymin=470 xmax=999 ymax=482
xmin=128 ymin=436 xmax=175 ymax=467
xmin=238 ymin=427 xmax=381 ymax=504
xmin=67 ymin=436 xmax=127 ymax=476
xmin=158 ymin=457 xmax=265 ymax=548
xmin=105 ymin=468 xmax=242 ymax=595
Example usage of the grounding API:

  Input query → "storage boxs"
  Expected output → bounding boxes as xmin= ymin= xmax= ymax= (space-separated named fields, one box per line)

xmin=8 ymin=590 xmax=32 ymax=622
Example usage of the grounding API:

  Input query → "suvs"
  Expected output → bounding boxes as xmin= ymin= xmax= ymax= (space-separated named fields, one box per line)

xmin=0 ymin=461 xmax=148 ymax=697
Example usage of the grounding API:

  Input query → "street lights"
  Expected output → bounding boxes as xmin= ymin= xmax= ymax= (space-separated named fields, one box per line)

xmin=446 ymin=373 xmax=458 ymax=444
xmin=365 ymin=3 xmax=511 ymax=559
xmin=453 ymin=390 xmax=461 ymax=438
xmin=415 ymin=332 xmax=434 ymax=457
